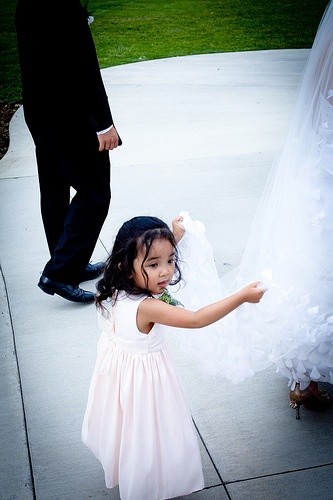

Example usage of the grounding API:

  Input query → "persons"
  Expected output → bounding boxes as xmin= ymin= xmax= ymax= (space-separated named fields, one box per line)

xmin=12 ymin=0 xmax=122 ymax=305
xmin=80 ymin=216 xmax=267 ymax=500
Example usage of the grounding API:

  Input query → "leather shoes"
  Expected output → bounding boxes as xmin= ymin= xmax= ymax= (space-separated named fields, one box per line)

xmin=78 ymin=262 xmax=106 ymax=283
xmin=38 ymin=274 xmax=95 ymax=302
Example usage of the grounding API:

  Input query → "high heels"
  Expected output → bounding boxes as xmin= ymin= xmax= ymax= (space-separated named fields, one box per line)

xmin=290 ymin=381 xmax=333 ymax=419
